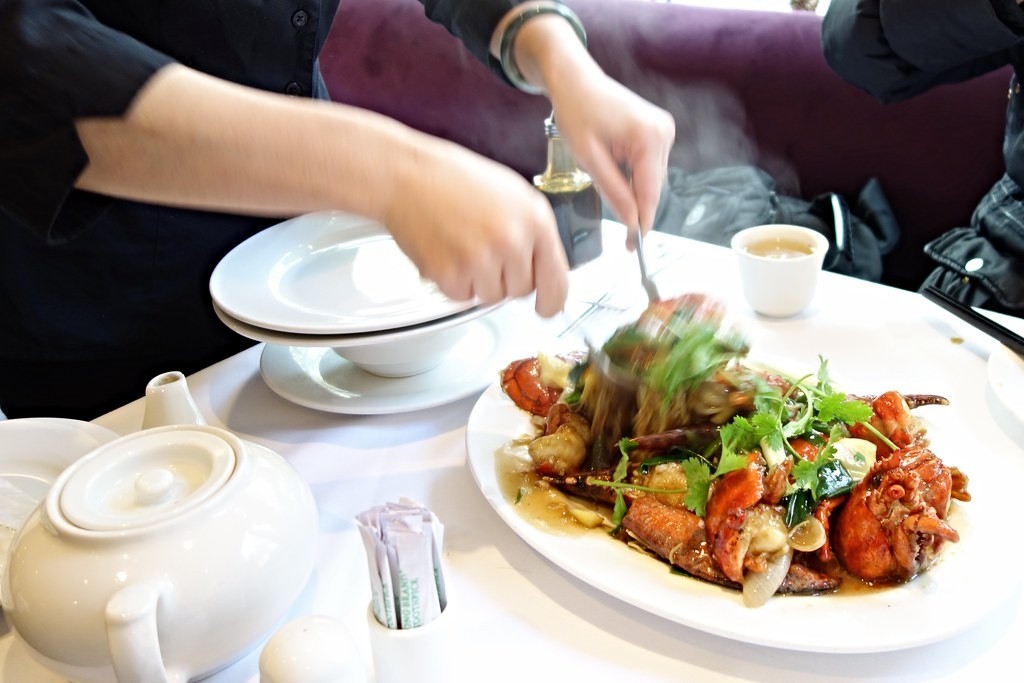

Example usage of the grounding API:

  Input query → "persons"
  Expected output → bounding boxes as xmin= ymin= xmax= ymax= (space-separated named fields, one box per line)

xmin=0 ymin=0 xmax=676 ymax=422
xmin=820 ymin=0 xmax=1024 ymax=317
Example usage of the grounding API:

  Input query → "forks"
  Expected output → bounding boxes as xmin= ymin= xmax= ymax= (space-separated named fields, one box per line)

xmin=618 ymin=161 xmax=663 ymax=306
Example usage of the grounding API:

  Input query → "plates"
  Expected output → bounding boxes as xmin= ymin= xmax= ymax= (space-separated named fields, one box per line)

xmin=256 ymin=314 xmax=527 ymax=415
xmin=207 ymin=214 xmax=520 ymax=350
xmin=467 ymin=317 xmax=1024 ymax=653
xmin=988 ymin=341 xmax=1024 ymax=422
xmin=0 ymin=417 xmax=123 ymax=570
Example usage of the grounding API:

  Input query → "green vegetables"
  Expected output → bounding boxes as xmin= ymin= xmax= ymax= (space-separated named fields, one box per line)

xmin=567 ymin=306 xmax=900 ymax=534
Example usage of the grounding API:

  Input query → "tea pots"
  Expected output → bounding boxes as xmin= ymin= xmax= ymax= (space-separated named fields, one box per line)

xmin=0 ymin=370 xmax=322 ymax=683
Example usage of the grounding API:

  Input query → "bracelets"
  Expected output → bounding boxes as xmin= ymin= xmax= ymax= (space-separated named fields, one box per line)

xmin=500 ymin=4 xmax=586 ymax=94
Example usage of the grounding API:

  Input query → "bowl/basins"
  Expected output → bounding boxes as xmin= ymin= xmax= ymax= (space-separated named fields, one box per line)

xmin=335 ymin=324 xmax=468 ymax=382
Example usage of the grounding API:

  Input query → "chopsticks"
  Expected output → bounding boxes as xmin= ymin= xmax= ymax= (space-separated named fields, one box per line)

xmin=923 ymin=284 xmax=1024 ymax=360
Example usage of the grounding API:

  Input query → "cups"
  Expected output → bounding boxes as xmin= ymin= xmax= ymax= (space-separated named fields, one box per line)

xmin=259 ymin=616 xmax=374 ymax=683
xmin=730 ymin=224 xmax=830 ymax=319
xmin=367 ymin=598 xmax=456 ymax=683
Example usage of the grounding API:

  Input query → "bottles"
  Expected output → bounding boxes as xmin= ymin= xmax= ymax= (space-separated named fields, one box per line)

xmin=537 ymin=118 xmax=603 ymax=274
